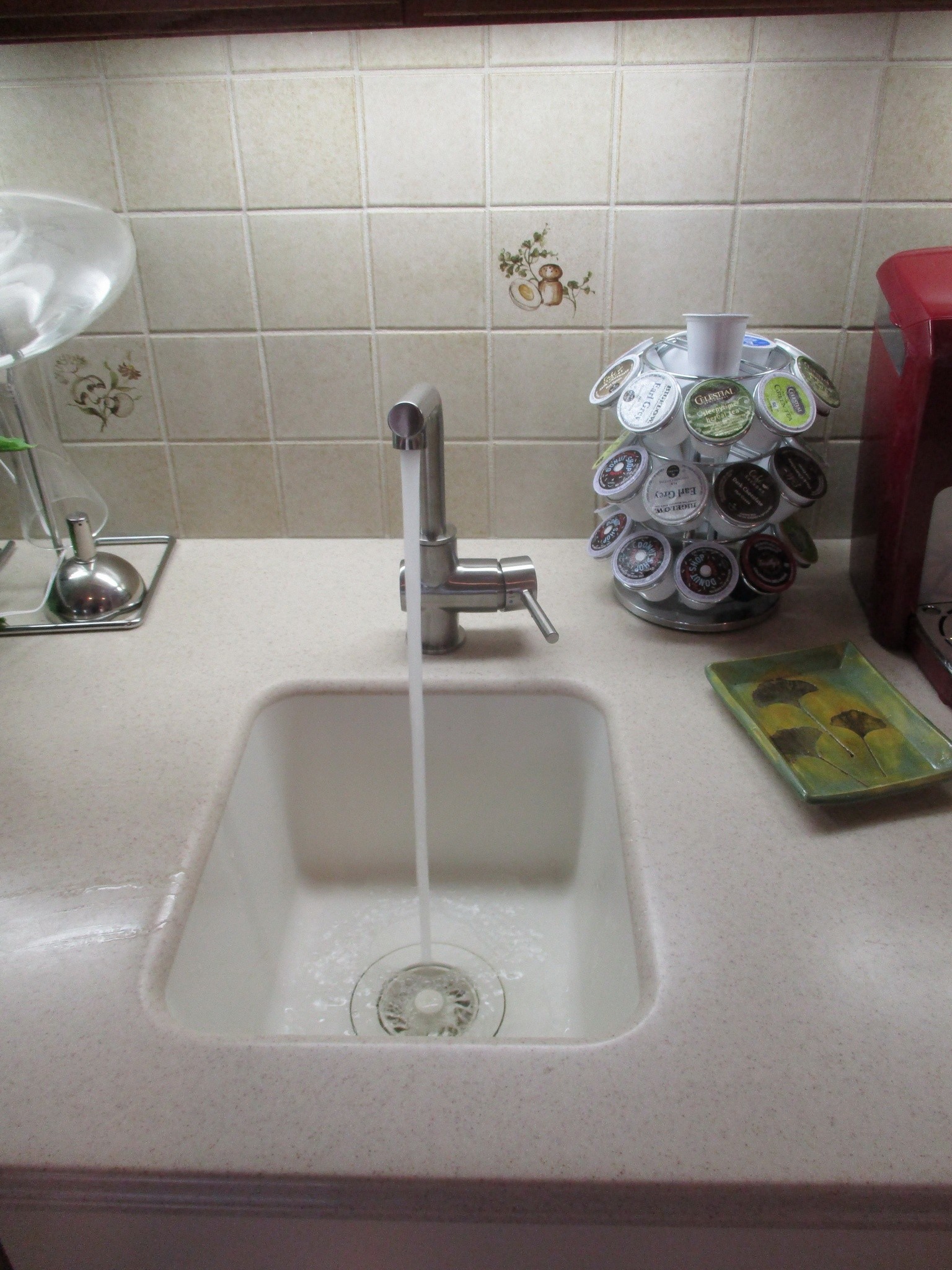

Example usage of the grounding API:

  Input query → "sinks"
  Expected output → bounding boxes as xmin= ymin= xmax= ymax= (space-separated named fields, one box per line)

xmin=139 ymin=674 xmax=669 ymax=1052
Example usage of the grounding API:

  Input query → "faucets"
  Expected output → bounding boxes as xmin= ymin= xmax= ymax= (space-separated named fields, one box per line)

xmin=379 ymin=382 xmax=562 ymax=658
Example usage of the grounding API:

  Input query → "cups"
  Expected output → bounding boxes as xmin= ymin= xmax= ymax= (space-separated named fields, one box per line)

xmin=588 ymin=310 xmax=842 ymax=612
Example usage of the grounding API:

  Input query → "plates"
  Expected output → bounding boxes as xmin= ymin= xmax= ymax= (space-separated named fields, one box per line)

xmin=704 ymin=641 xmax=952 ymax=807
